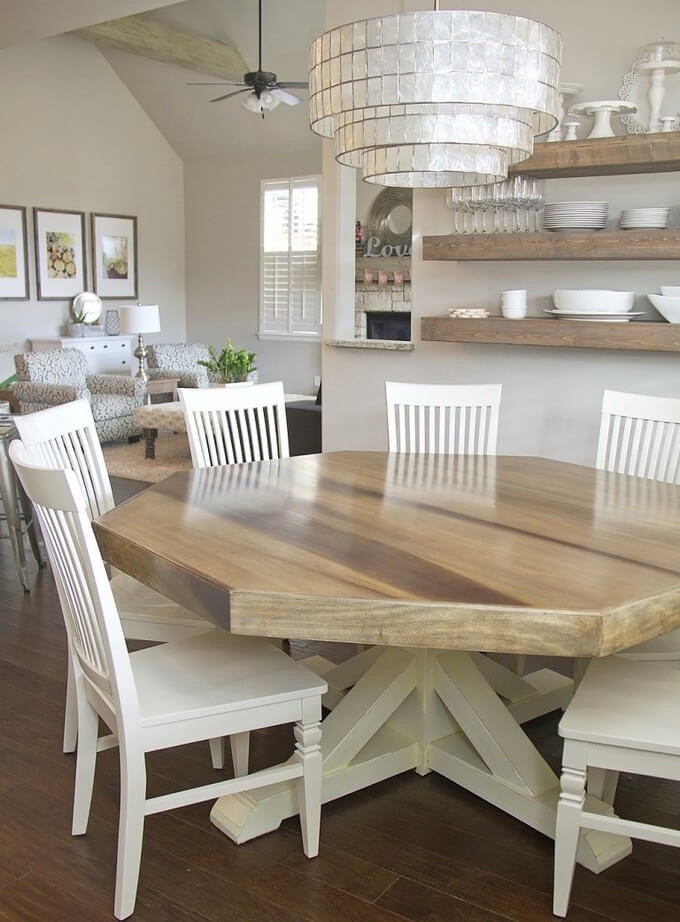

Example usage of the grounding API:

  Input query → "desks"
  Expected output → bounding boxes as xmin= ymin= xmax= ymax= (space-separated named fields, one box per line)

xmin=134 ymin=394 xmax=316 ymax=460
xmin=90 ymin=451 xmax=680 ymax=874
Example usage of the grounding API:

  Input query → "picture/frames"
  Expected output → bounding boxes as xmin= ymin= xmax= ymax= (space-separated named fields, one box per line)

xmin=90 ymin=212 xmax=138 ymax=301
xmin=32 ymin=206 xmax=88 ymax=301
xmin=0 ymin=204 xmax=31 ymax=302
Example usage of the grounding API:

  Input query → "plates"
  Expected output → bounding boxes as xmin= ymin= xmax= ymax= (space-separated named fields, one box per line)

xmin=618 ymin=207 xmax=672 ymax=230
xmin=544 ymin=201 xmax=609 ymax=232
xmin=542 ymin=309 xmax=646 ymax=323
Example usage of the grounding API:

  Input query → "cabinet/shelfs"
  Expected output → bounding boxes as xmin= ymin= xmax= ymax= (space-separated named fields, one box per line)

xmin=32 ymin=335 xmax=135 ymax=377
xmin=420 ymin=131 xmax=680 ymax=352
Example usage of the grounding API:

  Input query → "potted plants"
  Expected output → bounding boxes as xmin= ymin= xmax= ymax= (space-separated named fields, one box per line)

xmin=196 ymin=336 xmax=258 ymax=389
xmin=67 ymin=310 xmax=88 ymax=337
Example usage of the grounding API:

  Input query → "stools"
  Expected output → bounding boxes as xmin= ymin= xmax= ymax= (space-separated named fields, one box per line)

xmin=0 ymin=427 xmax=47 ymax=592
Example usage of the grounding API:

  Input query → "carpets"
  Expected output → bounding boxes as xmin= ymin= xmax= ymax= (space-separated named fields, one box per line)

xmin=102 ymin=430 xmax=193 ymax=482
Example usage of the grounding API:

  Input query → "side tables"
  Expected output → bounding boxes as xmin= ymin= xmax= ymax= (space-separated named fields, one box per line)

xmin=141 ymin=379 xmax=179 ymax=440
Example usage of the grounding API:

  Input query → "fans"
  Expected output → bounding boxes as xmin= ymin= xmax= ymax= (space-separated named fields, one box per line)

xmin=185 ymin=0 xmax=308 ymax=120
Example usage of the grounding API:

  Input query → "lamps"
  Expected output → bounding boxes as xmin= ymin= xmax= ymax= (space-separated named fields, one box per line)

xmin=118 ymin=302 xmax=160 ymax=381
xmin=307 ymin=0 xmax=563 ymax=188
xmin=241 ymin=88 xmax=300 ymax=113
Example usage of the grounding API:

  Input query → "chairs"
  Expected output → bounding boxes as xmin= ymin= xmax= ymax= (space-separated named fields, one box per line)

xmin=553 ymin=656 xmax=680 ymax=918
xmin=12 ymin=397 xmax=225 ymax=769
xmin=7 ymin=439 xmax=320 ymax=920
xmin=177 ymin=381 xmax=290 ymax=469
xmin=385 ymin=382 xmax=502 ymax=455
xmin=595 ymin=391 xmax=680 ymax=485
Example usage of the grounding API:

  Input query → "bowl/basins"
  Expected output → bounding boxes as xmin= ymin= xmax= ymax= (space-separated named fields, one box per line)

xmin=648 ymin=294 xmax=680 ymax=323
xmin=551 ymin=289 xmax=634 ymax=313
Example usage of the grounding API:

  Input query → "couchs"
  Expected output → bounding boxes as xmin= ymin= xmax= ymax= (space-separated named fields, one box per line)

xmin=144 ymin=343 xmax=210 ymax=388
xmin=14 ymin=348 xmax=143 ymax=447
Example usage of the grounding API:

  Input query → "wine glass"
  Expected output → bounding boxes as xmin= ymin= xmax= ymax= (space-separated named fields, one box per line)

xmin=444 ymin=174 xmax=546 ymax=235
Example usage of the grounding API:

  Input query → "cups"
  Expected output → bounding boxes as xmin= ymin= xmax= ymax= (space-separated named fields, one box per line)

xmin=500 ymin=289 xmax=529 ymax=320
xmin=661 ymin=286 xmax=680 ymax=297
